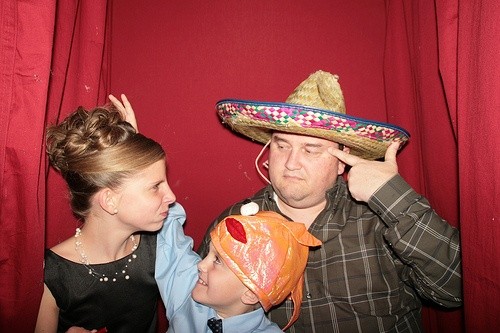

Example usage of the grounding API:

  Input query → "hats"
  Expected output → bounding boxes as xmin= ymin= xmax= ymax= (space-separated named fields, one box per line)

xmin=215 ymin=69 xmax=411 ymax=160
xmin=210 ymin=199 xmax=323 ymax=331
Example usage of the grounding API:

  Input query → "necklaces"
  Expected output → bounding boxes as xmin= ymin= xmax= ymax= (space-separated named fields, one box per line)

xmin=74 ymin=226 xmax=138 ymax=283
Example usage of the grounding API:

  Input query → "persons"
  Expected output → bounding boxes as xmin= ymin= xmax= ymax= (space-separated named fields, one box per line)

xmin=108 ymin=94 xmax=323 ymax=333
xmin=195 ymin=68 xmax=463 ymax=333
xmin=34 ymin=104 xmax=175 ymax=333
